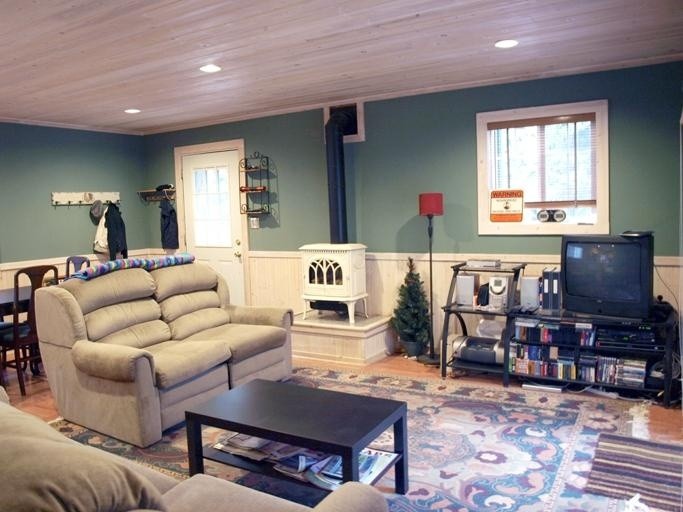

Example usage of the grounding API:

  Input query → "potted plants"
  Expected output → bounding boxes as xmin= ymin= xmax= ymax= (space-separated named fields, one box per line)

xmin=390 ymin=254 xmax=431 ymax=357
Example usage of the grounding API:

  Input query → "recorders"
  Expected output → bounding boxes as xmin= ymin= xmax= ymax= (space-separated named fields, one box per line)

xmin=452 ymin=335 xmax=504 ymax=364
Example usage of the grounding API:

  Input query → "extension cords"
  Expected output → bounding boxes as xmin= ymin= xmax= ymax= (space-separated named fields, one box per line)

xmin=585 ymin=386 xmax=620 ymax=399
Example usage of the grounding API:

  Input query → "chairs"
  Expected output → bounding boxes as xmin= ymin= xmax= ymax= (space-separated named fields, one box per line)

xmin=0 ymin=255 xmax=89 ymax=398
xmin=0 ymin=389 xmax=391 ymax=511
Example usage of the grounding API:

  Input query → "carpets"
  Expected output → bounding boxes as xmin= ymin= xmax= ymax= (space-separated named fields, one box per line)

xmin=40 ymin=367 xmax=652 ymax=512
xmin=585 ymin=432 xmax=681 ymax=512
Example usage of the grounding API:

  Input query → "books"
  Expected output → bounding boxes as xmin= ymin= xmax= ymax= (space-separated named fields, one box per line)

xmin=467 ymin=259 xmax=501 ymax=267
xmin=542 ymin=267 xmax=561 ymax=309
xmin=213 ymin=433 xmax=400 ymax=491
xmin=509 ymin=317 xmax=646 ymax=393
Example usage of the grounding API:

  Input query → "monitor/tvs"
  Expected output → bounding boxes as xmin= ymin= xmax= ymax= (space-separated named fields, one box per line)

xmin=560 ymin=233 xmax=654 ymax=319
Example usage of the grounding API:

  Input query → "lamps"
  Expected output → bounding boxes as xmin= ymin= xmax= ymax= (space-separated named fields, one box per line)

xmin=417 ymin=193 xmax=444 ymax=362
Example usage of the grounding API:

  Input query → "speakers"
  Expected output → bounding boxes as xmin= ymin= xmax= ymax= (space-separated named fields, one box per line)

xmin=456 ymin=273 xmax=480 ymax=305
xmin=520 ymin=276 xmax=542 ymax=307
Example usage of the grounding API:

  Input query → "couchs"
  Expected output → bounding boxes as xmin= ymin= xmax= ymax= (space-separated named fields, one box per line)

xmin=34 ymin=262 xmax=294 ymax=449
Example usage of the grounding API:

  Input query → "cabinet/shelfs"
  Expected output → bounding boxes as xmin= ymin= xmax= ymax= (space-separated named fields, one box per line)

xmin=236 ymin=151 xmax=271 ymax=218
xmin=439 ymin=261 xmax=679 ymax=410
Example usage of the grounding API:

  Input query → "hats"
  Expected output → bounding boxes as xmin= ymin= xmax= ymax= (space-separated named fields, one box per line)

xmin=89 ymin=199 xmax=104 ymax=225
